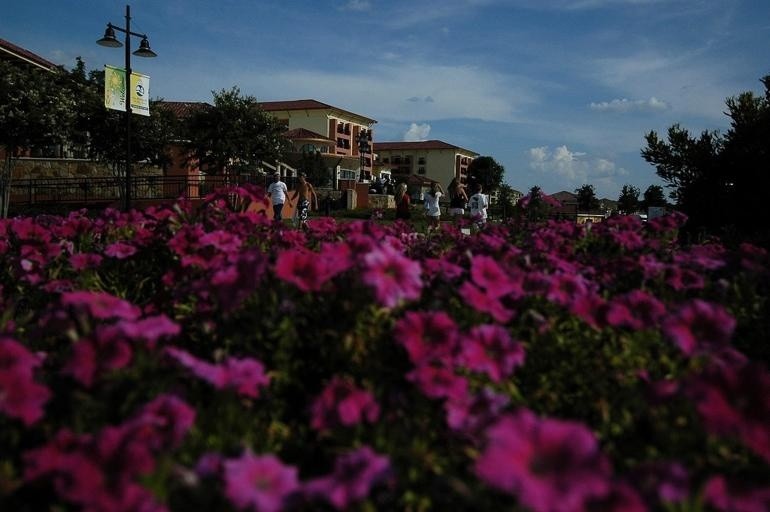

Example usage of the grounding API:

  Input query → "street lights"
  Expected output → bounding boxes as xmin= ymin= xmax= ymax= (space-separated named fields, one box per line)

xmin=96 ymin=4 xmax=158 ymax=210
xmin=355 ymin=129 xmax=372 ymax=178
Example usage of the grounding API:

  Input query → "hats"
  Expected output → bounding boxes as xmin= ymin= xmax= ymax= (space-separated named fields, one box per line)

xmin=273 ymin=172 xmax=280 ymax=176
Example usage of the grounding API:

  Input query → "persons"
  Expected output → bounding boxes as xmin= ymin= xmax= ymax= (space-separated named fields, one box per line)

xmin=423 ymin=181 xmax=445 ymax=232
xmin=291 ymin=172 xmax=318 ymax=232
xmin=393 ymin=183 xmax=417 ymax=220
xmin=263 ymin=172 xmax=294 ymax=222
xmin=466 ymin=183 xmax=489 ymax=224
xmin=446 ymin=178 xmax=468 ymax=215
